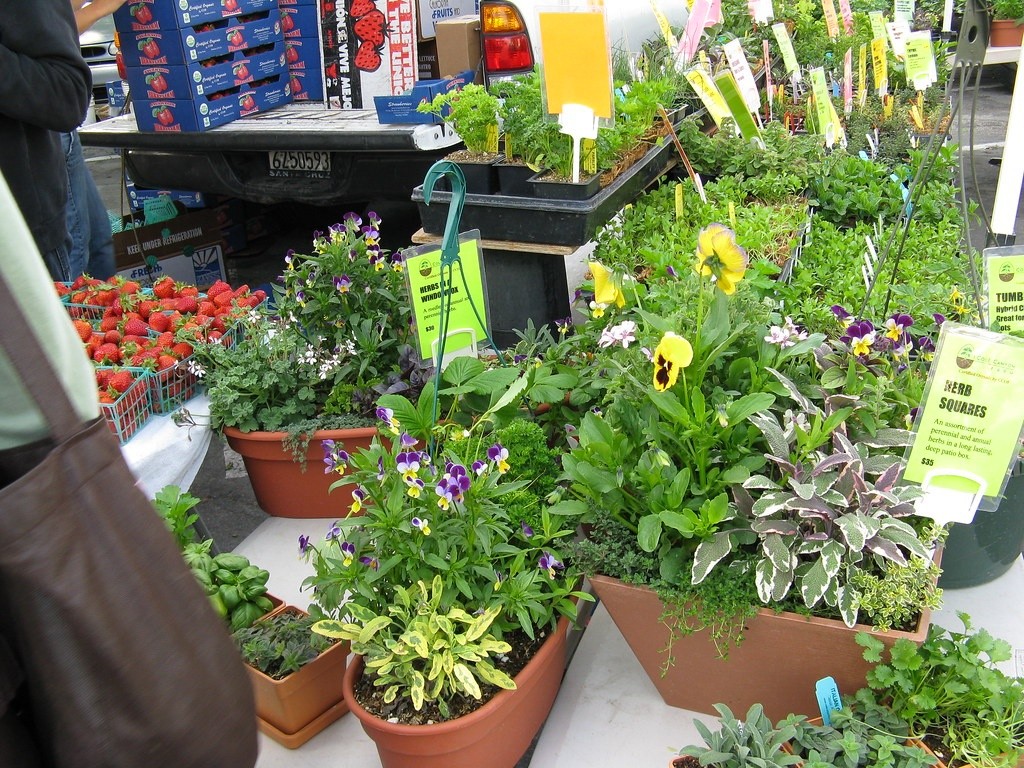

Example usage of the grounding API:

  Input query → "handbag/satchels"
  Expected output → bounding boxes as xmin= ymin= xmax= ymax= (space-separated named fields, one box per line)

xmin=1 ymin=281 xmax=258 ymax=768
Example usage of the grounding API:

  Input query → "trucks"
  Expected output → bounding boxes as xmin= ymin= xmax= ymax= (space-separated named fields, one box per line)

xmin=77 ymin=0 xmax=702 ymax=256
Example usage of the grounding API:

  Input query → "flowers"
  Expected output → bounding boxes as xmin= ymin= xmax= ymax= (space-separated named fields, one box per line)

xmin=168 ymin=200 xmax=982 ymax=719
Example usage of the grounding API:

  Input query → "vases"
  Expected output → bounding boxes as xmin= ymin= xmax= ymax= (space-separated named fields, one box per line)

xmin=341 ymin=568 xmax=583 ymax=768
xmin=223 ymin=421 xmax=452 ymax=519
xmin=588 ymin=539 xmax=945 ymax=729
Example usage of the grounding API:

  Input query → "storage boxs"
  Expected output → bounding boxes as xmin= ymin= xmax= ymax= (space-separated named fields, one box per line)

xmin=106 ymin=0 xmax=481 ymax=131
xmin=410 ymin=134 xmax=674 ymax=246
xmin=112 ymin=167 xmax=232 ymax=293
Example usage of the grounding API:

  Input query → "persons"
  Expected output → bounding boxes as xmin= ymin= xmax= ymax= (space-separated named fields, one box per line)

xmin=0 ymin=0 xmax=124 ymax=768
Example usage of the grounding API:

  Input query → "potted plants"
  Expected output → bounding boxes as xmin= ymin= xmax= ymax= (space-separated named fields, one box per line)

xmin=671 ymin=611 xmax=1023 ymax=767
xmin=152 ymin=485 xmax=351 ymax=736
xmin=419 ymin=0 xmax=1024 ymax=269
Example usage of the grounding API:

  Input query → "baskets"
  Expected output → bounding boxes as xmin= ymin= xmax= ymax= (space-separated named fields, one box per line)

xmin=50 ymin=272 xmax=269 ymax=448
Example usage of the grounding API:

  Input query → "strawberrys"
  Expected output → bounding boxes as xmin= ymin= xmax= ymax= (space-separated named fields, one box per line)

xmin=195 ymin=15 xmax=271 ymax=100
xmin=54 ymin=276 xmax=266 ymax=440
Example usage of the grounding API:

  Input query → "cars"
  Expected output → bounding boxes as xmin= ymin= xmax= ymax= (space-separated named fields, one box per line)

xmin=70 ymin=0 xmax=130 ymax=105
